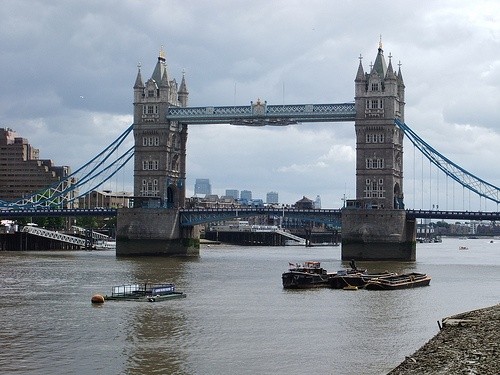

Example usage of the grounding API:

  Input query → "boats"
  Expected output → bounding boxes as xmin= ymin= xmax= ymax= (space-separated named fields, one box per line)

xmin=328 ymin=257 xmax=390 ymax=290
xmin=282 ymin=261 xmax=337 ymax=287
xmin=364 ymin=272 xmax=431 ymax=290
